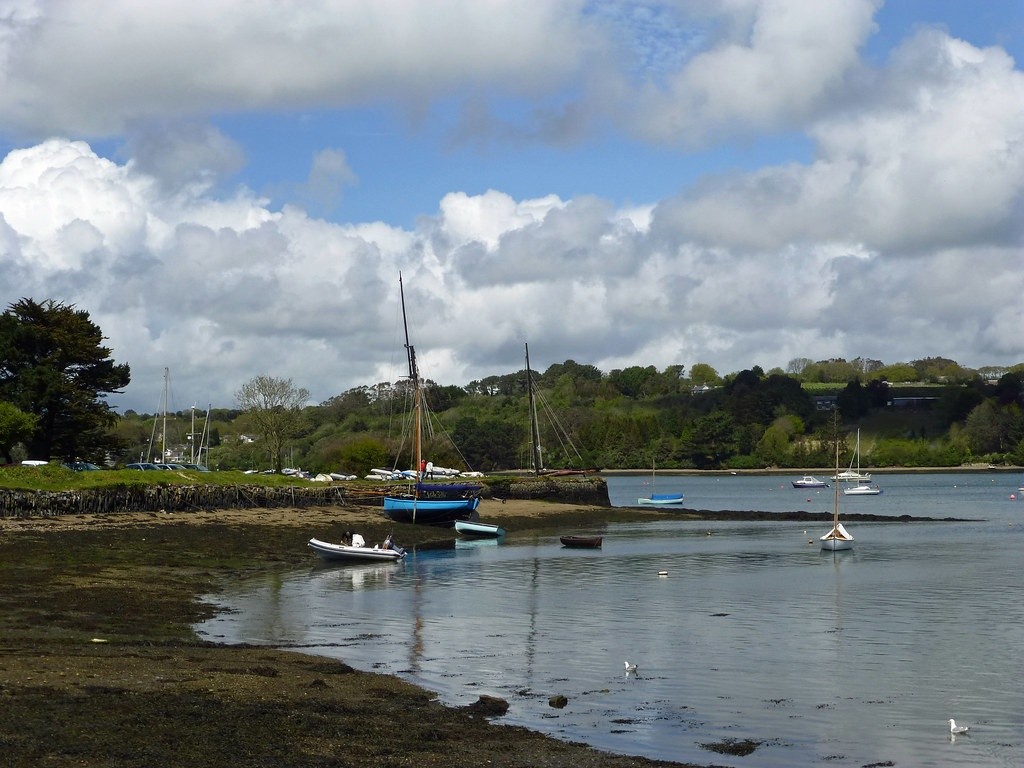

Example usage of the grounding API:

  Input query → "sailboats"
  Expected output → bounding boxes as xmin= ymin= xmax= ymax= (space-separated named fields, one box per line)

xmin=636 ymin=459 xmax=684 ymax=505
xmin=819 ymin=441 xmax=855 ymax=552
xmin=381 ymin=270 xmax=481 ymax=525
xmin=521 ymin=343 xmax=605 ymax=477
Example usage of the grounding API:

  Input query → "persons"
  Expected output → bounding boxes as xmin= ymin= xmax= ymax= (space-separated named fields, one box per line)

xmin=425 ymin=460 xmax=434 ymax=480
xmin=341 ymin=529 xmax=355 ymax=546
xmin=420 ymin=459 xmax=426 ymax=480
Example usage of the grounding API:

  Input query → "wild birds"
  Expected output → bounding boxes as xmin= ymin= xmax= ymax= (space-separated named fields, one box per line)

xmin=624 ymin=660 xmax=638 ymax=672
xmin=946 ymin=717 xmax=971 ymax=735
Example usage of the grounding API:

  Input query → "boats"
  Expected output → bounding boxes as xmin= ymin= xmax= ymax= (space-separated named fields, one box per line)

xmin=560 ymin=535 xmax=604 ymax=547
xmin=308 ymin=538 xmax=407 ymax=560
xmin=454 ymin=520 xmax=506 ymax=537
xmin=792 ymin=428 xmax=880 ymax=495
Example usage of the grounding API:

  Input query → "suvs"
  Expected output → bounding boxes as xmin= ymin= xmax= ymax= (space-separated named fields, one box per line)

xmin=126 ymin=463 xmax=160 ymax=471
xmin=67 ymin=463 xmax=101 ymax=471
xmin=155 ymin=464 xmax=187 ymax=470
xmin=182 ymin=464 xmax=211 ymax=473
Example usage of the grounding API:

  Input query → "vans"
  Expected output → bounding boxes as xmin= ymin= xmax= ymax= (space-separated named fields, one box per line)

xmin=21 ymin=460 xmax=49 ymax=467
